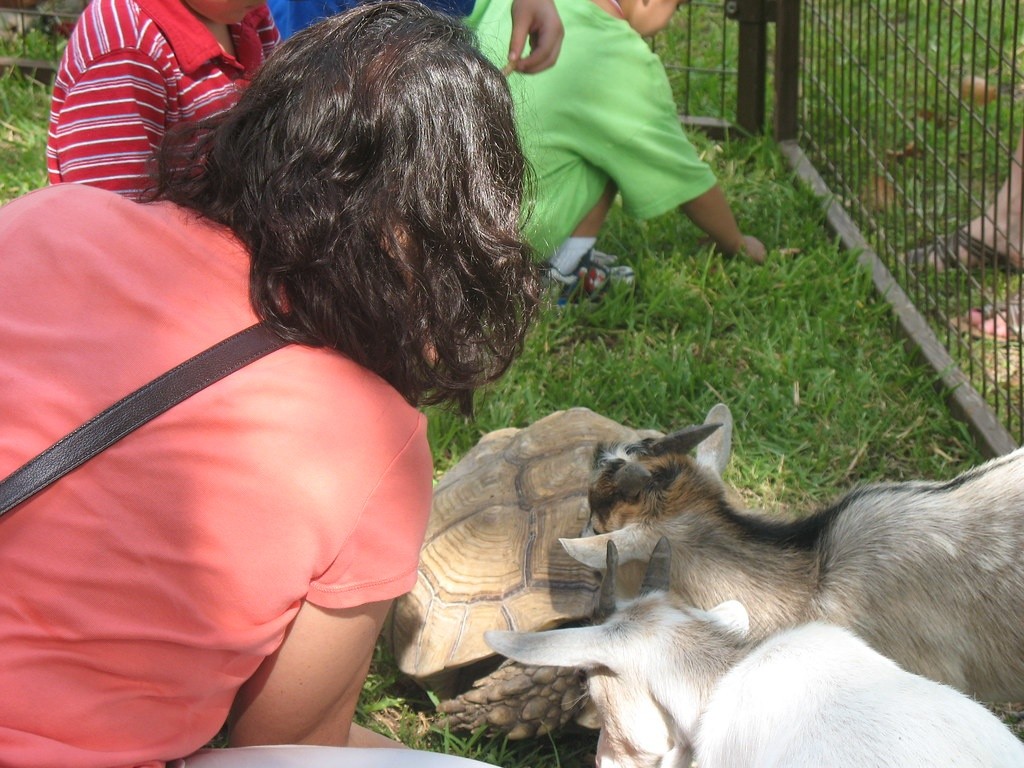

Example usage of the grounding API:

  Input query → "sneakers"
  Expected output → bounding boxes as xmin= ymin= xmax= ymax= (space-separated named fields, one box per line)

xmin=538 ymin=247 xmax=635 ymax=315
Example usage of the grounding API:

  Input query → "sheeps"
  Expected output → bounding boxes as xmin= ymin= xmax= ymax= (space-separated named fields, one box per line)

xmin=556 ymin=404 xmax=1023 ymax=742
xmin=480 ymin=535 xmax=1024 ymax=768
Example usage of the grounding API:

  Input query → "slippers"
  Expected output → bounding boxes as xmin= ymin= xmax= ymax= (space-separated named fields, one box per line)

xmin=898 ymin=224 xmax=1024 ymax=274
xmin=951 ymin=288 xmax=1024 ymax=343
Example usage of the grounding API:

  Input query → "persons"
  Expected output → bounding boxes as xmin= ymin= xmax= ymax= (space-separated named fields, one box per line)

xmin=898 ymin=125 xmax=1020 ymax=345
xmin=1 ymin=0 xmax=545 ymax=767
xmin=44 ymin=0 xmax=765 ymax=311
